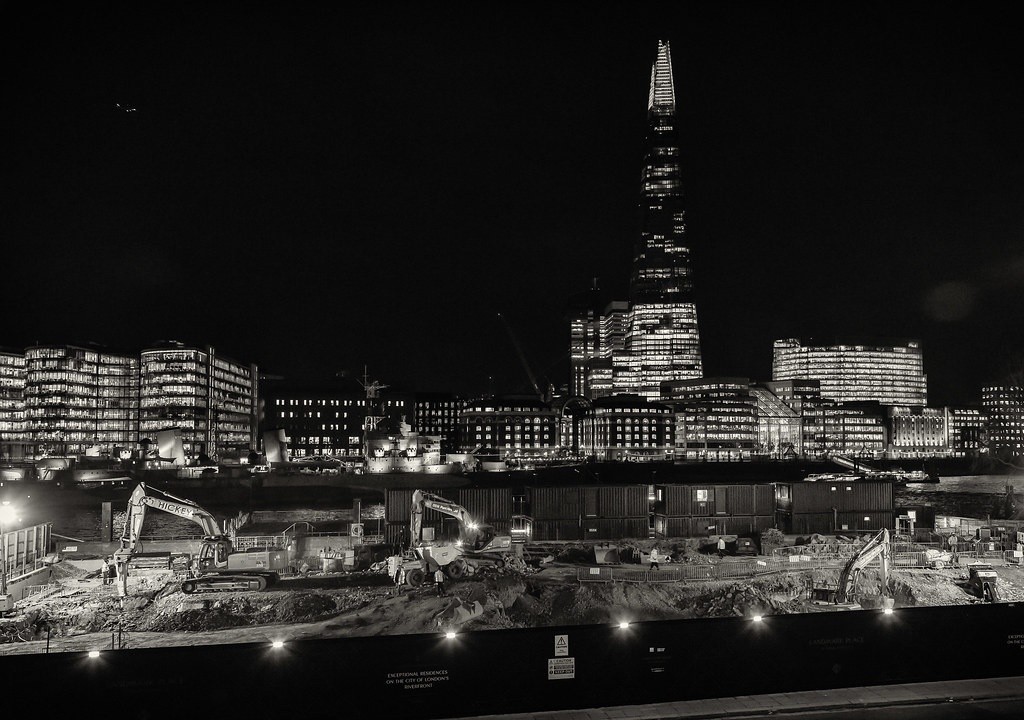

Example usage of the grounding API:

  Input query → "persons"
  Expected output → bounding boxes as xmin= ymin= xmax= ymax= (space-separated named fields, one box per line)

xmin=717 ymin=537 xmax=725 ymax=559
xmin=948 ymin=532 xmax=958 ymax=553
xmin=393 ymin=564 xmax=405 ymax=597
xmin=102 ymin=559 xmax=109 ymax=585
xmin=434 ymin=566 xmax=448 ymax=598
xmin=649 ymin=545 xmax=659 ymax=570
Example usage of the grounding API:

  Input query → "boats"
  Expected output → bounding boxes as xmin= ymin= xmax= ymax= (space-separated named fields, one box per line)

xmin=239 ymin=415 xmax=596 ymax=510
xmin=803 ymin=468 xmax=940 ymax=487
xmin=0 ymin=437 xmax=183 ymax=508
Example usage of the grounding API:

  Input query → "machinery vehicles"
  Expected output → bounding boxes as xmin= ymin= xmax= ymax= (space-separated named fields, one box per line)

xmin=966 ymin=561 xmax=1008 ymax=604
xmin=387 ymin=487 xmax=512 ymax=589
xmin=473 ymin=513 xmax=555 ymax=568
xmin=107 ymin=482 xmax=288 ymax=609
xmin=802 ymin=528 xmax=895 ymax=613
